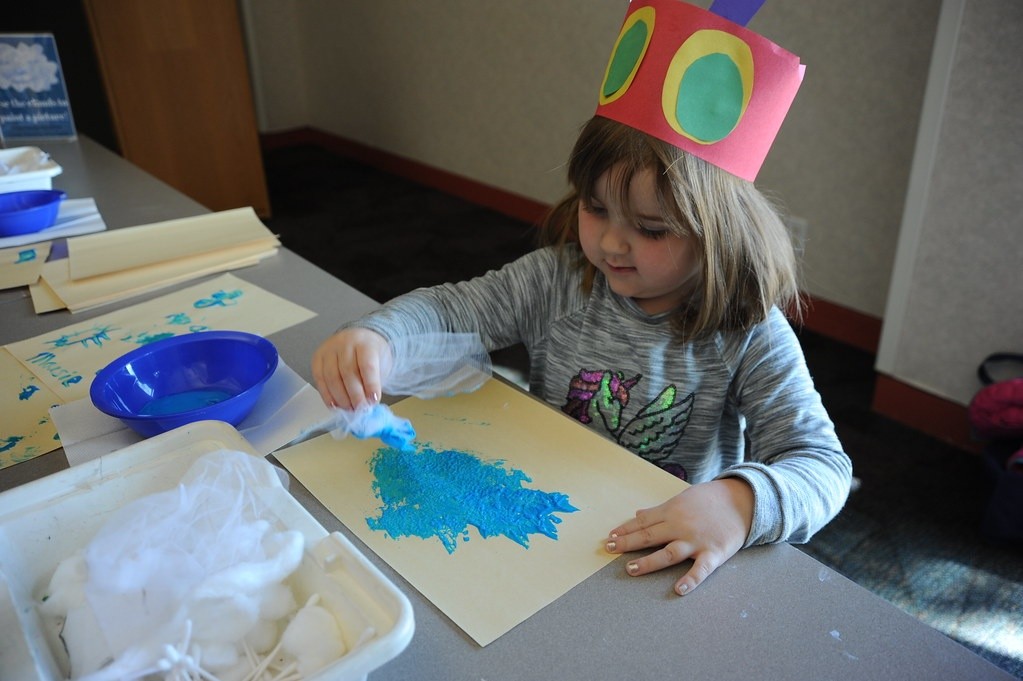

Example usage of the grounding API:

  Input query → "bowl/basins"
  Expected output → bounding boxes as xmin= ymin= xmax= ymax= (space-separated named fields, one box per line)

xmin=89 ymin=331 xmax=279 ymax=441
xmin=0 ymin=188 xmax=69 ymax=239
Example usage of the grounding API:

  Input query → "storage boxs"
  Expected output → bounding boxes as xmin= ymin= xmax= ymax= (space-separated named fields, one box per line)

xmin=0 ymin=417 xmax=419 ymax=681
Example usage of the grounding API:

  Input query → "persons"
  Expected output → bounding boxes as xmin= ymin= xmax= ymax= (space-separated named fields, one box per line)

xmin=315 ymin=3 xmax=854 ymax=596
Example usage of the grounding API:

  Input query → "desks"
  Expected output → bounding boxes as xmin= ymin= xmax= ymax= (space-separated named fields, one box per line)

xmin=0 ymin=134 xmax=1015 ymax=679
